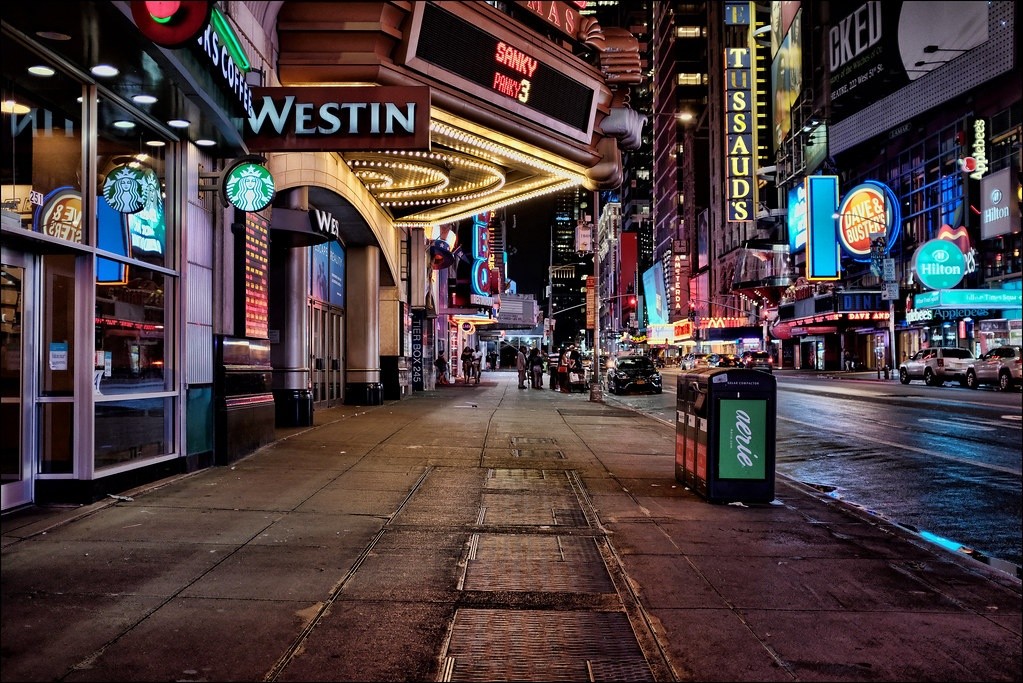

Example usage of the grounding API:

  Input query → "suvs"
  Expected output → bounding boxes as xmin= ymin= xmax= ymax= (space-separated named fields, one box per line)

xmin=899 ymin=347 xmax=976 ymax=387
xmin=738 ymin=351 xmax=773 ymax=374
xmin=967 ymin=345 xmax=1023 ymax=390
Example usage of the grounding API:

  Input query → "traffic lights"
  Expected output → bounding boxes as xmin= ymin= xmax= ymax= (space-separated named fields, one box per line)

xmin=688 ymin=302 xmax=696 ymax=322
xmin=632 ymin=297 xmax=636 ymax=304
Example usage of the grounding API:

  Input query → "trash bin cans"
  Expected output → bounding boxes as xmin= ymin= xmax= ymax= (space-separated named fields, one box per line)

xmin=674 ymin=365 xmax=777 ymax=501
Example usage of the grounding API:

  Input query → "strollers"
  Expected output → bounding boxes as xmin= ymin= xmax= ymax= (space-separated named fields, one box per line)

xmin=569 ymin=361 xmax=588 ymax=394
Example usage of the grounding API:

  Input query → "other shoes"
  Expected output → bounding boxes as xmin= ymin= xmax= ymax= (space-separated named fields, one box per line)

xmin=518 ymin=385 xmax=528 ymax=389
xmin=437 ymin=380 xmax=445 ymax=385
xmin=464 ymin=380 xmax=470 ymax=384
xmin=475 ymin=380 xmax=481 ymax=384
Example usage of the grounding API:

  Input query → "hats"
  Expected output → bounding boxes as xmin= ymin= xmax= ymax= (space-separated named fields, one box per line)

xmin=569 ymin=345 xmax=574 ymax=348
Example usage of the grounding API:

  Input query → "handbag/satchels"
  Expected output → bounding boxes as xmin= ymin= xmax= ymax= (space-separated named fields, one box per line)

xmin=558 ymin=367 xmax=567 ymax=372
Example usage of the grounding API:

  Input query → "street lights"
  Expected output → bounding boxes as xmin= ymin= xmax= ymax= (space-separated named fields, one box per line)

xmin=608 ymin=109 xmax=694 ymax=351
xmin=832 ymin=211 xmax=898 ymax=380
xmin=670 ymin=286 xmax=701 ymax=352
xmin=547 ymin=262 xmax=586 ymax=352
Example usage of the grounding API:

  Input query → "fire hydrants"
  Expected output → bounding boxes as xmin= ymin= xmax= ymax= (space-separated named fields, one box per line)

xmin=883 ymin=366 xmax=890 ymax=380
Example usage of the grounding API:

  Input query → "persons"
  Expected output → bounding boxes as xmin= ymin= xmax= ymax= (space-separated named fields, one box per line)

xmin=461 ymin=345 xmax=483 ymax=384
xmin=436 ymin=350 xmax=447 ymax=384
xmin=901 ymin=351 xmax=916 ymax=363
xmin=516 ymin=345 xmax=583 ymax=394
xmin=844 ymin=351 xmax=862 ymax=372
xmin=486 ymin=351 xmax=499 ymax=371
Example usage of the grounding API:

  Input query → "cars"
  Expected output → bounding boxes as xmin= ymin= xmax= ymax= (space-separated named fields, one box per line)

xmin=599 ymin=355 xmax=614 ymax=372
xmin=548 ymin=352 xmax=561 ymax=371
xmin=682 ymin=353 xmax=709 ymax=370
xmin=607 ymin=355 xmax=662 ymax=394
xmin=582 ymin=357 xmax=593 ymax=368
xmin=562 ymin=350 xmax=571 ymax=366
xmin=696 ymin=352 xmax=742 ymax=368
xmin=653 ymin=358 xmax=666 ymax=368
